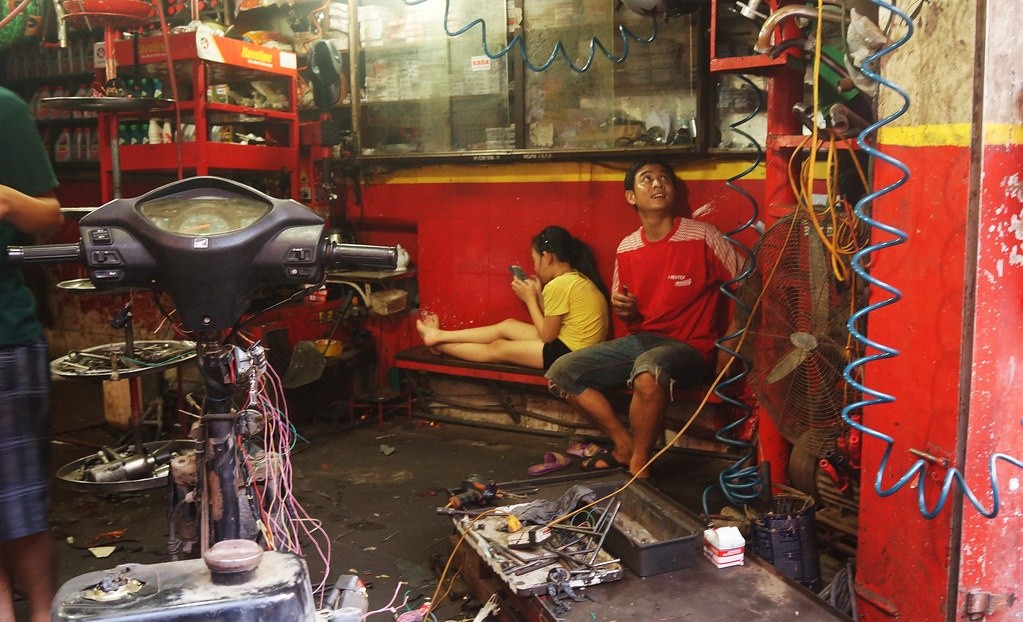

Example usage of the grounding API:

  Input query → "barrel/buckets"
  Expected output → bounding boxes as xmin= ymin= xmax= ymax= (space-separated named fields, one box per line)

xmin=746 ymin=485 xmax=822 ymax=593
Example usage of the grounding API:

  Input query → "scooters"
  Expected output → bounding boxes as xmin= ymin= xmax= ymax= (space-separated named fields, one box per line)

xmin=7 ymin=175 xmax=418 ymax=621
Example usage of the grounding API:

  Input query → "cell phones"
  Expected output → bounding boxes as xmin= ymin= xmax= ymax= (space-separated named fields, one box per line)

xmin=509 ymin=265 xmax=529 ymax=282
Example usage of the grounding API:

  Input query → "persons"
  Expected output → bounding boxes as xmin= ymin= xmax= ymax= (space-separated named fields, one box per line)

xmin=416 ymin=225 xmax=610 ymax=371
xmin=544 ymin=158 xmax=756 ymax=489
xmin=0 ymin=88 xmax=62 ymax=622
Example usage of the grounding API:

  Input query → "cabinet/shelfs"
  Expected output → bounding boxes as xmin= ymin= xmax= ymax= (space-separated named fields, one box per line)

xmin=0 ymin=30 xmax=299 ymax=206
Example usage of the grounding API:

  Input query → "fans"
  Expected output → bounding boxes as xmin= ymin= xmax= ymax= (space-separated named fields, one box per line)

xmin=736 ymin=215 xmax=866 ymax=460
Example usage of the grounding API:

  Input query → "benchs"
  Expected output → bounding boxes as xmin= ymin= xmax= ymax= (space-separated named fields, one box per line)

xmin=393 ymin=346 xmax=745 ymax=460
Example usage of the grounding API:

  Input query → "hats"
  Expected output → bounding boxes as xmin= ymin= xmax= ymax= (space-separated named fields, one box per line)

xmin=309 ymin=43 xmax=343 ymax=107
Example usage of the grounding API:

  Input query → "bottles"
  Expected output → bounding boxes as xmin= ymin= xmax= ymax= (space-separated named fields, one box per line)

xmin=0 ymin=37 xmax=174 ymax=162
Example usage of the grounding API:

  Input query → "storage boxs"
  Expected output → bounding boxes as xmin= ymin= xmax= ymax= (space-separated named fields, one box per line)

xmin=702 ymin=525 xmax=746 ymax=569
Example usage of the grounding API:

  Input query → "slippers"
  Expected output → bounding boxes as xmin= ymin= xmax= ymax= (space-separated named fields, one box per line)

xmin=578 ymin=448 xmax=628 ymax=471
xmin=566 ymin=443 xmax=608 ymax=458
xmin=528 ymin=451 xmax=572 ymax=475
